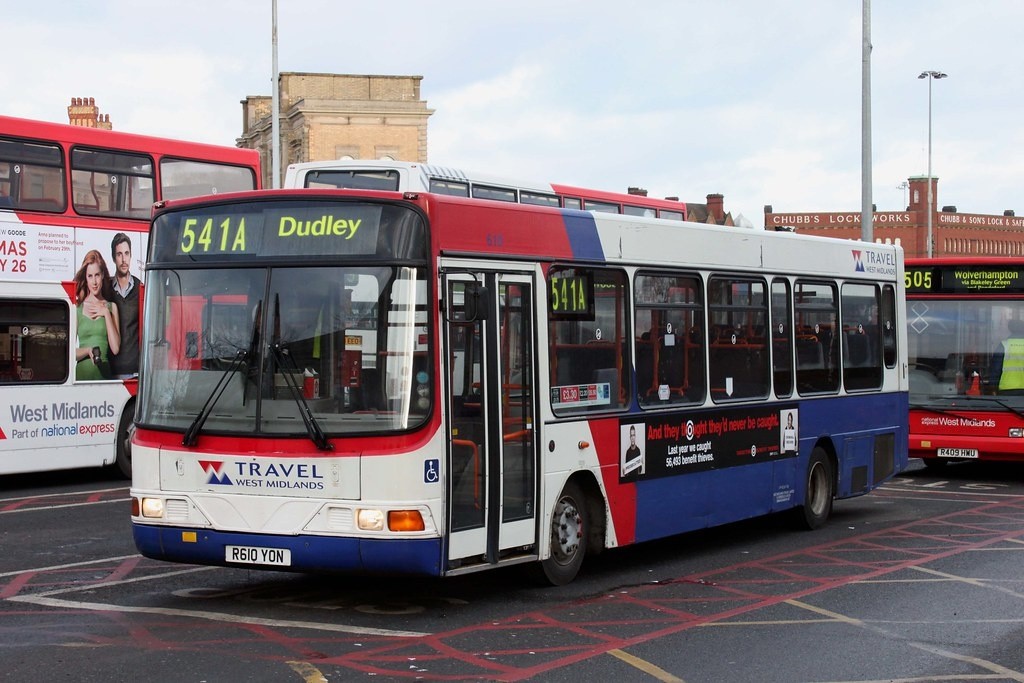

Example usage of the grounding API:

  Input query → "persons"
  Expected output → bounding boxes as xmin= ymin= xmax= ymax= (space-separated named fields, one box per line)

xmin=73 ymin=232 xmax=143 ymax=381
xmin=238 ymin=303 xmax=306 ymax=373
xmin=624 ymin=425 xmax=642 ymax=478
xmin=988 ymin=320 xmax=1024 ymax=397
xmin=782 ymin=412 xmax=797 ymax=453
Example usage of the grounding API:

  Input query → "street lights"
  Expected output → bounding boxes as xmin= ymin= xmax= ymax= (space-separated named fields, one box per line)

xmin=918 ymin=70 xmax=948 ymax=261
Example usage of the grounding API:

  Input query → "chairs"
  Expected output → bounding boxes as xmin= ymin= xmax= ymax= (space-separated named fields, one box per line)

xmin=471 ymin=322 xmax=880 ymax=415
xmin=1 ymin=195 xmax=98 ymax=215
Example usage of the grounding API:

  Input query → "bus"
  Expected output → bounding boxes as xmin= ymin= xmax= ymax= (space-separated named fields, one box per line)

xmin=125 ymin=186 xmax=909 ymax=588
xmin=280 ymin=156 xmax=689 ymax=423
xmin=847 ymin=256 xmax=1024 ymax=482
xmin=0 ymin=117 xmax=265 ymax=479
